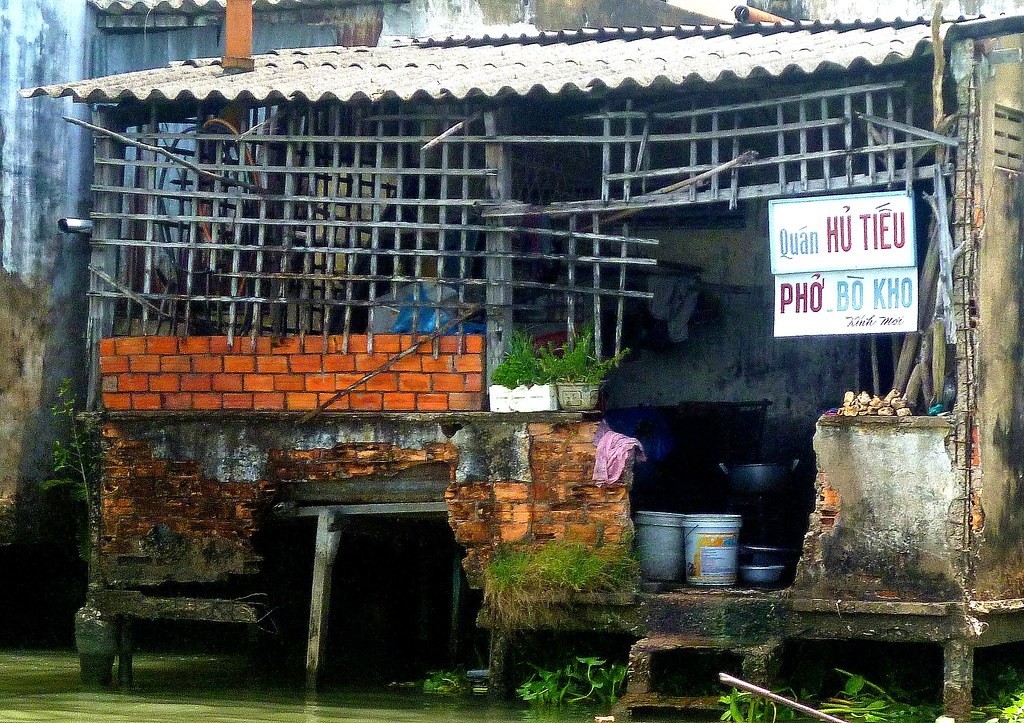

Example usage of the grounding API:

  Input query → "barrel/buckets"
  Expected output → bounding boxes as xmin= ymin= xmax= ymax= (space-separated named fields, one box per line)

xmin=682 ymin=514 xmax=742 ymax=586
xmin=635 ymin=511 xmax=684 ymax=580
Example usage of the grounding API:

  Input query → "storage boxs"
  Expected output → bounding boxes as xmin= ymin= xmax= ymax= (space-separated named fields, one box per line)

xmin=374 ymin=282 xmax=457 ymax=333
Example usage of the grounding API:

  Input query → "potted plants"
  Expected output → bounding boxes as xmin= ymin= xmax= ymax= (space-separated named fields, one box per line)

xmin=490 ymin=332 xmax=558 ymax=414
xmin=543 ymin=325 xmax=631 ymax=410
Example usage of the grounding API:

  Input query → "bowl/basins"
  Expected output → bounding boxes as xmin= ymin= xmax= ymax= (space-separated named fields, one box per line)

xmin=740 ymin=566 xmax=785 ymax=585
xmin=743 ymin=545 xmax=797 ymax=567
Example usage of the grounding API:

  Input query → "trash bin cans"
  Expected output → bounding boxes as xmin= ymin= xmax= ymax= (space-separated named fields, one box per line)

xmin=683 ymin=515 xmax=742 ymax=586
xmin=635 ymin=510 xmax=684 ymax=583
